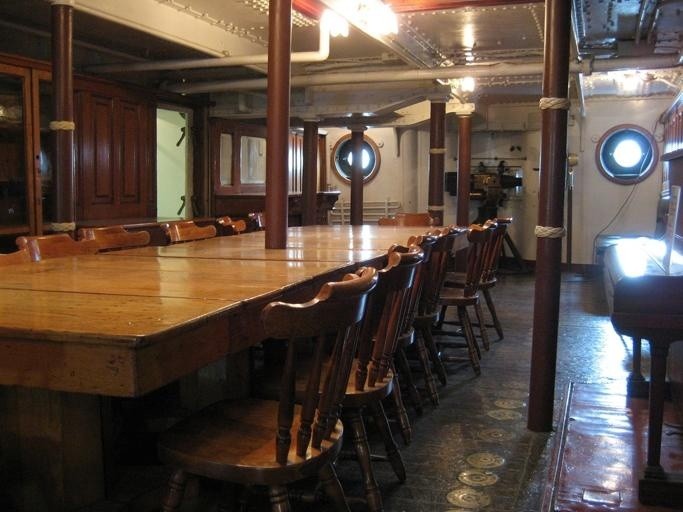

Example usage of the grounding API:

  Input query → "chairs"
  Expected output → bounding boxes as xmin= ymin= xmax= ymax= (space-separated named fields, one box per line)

xmin=369 ymin=235 xmax=440 ymax=444
xmin=158 ymin=266 xmax=379 ymax=512
xmin=265 ymin=243 xmax=425 ymax=512
xmin=161 ymin=221 xmax=217 ymax=243
xmin=436 ymin=215 xmax=513 ymax=349
xmin=431 ymin=219 xmax=498 ymax=386
xmin=15 ymin=234 xmax=100 ymax=262
xmin=395 ymin=227 xmax=458 ymax=416
xmin=0 ymin=248 xmax=29 ymax=268
xmin=78 ymin=225 xmax=151 ymax=251
xmin=216 ymin=216 xmax=246 ymax=234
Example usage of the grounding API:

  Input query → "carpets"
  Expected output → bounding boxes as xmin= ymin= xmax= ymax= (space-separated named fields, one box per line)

xmin=536 ymin=382 xmax=683 ymax=512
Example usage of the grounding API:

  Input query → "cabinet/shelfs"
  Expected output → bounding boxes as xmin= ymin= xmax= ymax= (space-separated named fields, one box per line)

xmin=75 ymin=93 xmax=158 ymax=227
xmin=0 ymin=53 xmax=56 ymax=234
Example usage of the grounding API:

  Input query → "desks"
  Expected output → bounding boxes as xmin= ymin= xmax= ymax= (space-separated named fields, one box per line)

xmin=605 ymin=237 xmax=683 ymax=510
xmin=0 ymin=225 xmax=461 ymax=511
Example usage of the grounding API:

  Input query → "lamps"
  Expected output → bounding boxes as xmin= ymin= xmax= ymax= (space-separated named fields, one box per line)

xmin=461 ymin=77 xmax=475 ymax=101
